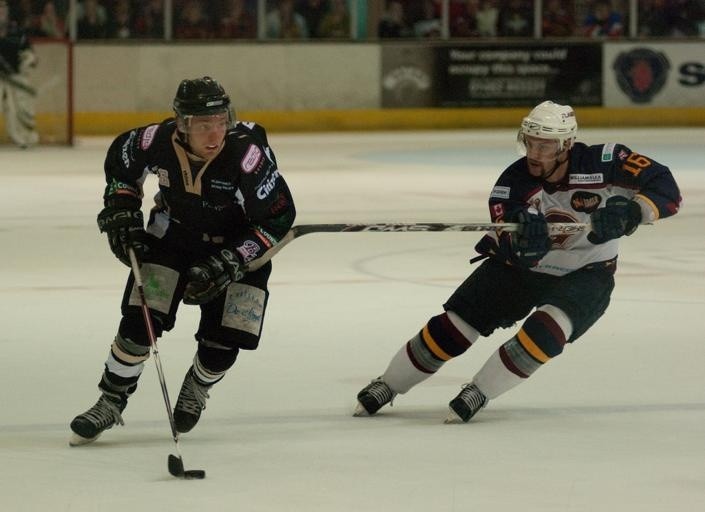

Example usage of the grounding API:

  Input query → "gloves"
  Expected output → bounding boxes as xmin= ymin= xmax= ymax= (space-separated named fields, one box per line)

xmin=509 ymin=208 xmax=552 ymax=267
xmin=97 ymin=206 xmax=146 ymax=268
xmin=181 ymin=249 xmax=246 ymax=304
xmin=587 ymin=195 xmax=641 ymax=245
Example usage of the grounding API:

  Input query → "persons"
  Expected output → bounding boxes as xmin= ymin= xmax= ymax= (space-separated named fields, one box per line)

xmin=379 ymin=1 xmax=703 ymax=38
xmin=357 ymin=97 xmax=683 ymax=427
xmin=11 ymin=1 xmax=351 ymax=37
xmin=0 ymin=3 xmax=42 ymax=148
xmin=68 ymin=75 xmax=297 ymax=438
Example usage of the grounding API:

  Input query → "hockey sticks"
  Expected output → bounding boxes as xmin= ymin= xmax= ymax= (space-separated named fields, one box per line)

xmin=129 ymin=249 xmax=205 ymax=478
xmin=243 ymin=223 xmax=592 ymax=272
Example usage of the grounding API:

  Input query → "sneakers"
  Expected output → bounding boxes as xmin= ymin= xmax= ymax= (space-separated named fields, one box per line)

xmin=174 ymin=366 xmax=225 ymax=434
xmin=357 ymin=375 xmax=395 ymax=414
xmin=71 ymin=389 xmax=128 ymax=438
xmin=448 ymin=383 xmax=486 ymax=423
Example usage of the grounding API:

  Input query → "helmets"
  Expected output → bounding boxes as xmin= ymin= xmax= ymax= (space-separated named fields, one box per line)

xmin=516 ymin=101 xmax=577 ymax=162
xmin=172 ymin=76 xmax=236 ymax=134
